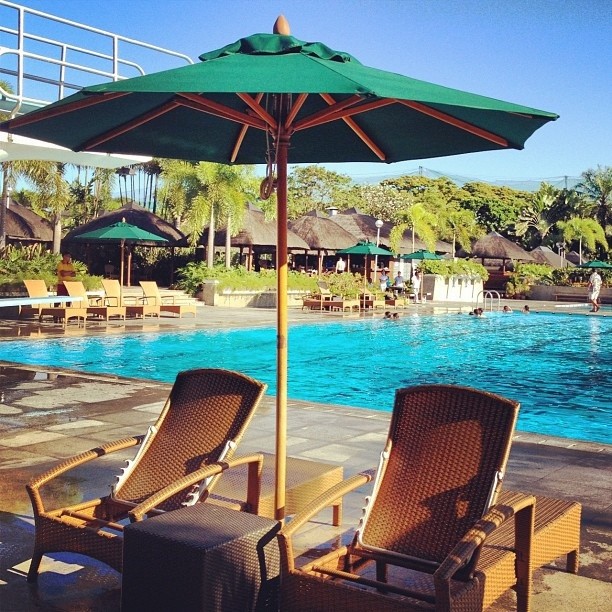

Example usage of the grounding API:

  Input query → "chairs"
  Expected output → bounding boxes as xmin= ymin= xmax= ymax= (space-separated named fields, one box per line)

xmin=25 ymin=368 xmax=344 ymax=583
xmin=278 ymin=384 xmax=584 ymax=612
xmin=302 ymin=294 xmax=320 ymax=311
xmin=316 ymin=280 xmax=361 ymax=316
xmin=386 ymin=290 xmax=404 ymax=308
xmin=360 ymin=287 xmax=386 ymax=313
xmin=62 ymin=280 xmax=126 ymax=321
xmin=100 ymin=279 xmax=160 ymax=320
xmin=21 ymin=280 xmax=87 ymax=331
xmin=138 ymin=280 xmax=197 ymax=319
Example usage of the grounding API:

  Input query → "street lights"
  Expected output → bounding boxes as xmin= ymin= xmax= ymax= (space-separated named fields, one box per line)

xmin=374 ymin=220 xmax=384 ymax=282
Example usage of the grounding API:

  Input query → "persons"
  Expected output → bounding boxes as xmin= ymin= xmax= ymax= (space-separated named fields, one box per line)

xmin=335 ymin=257 xmax=346 ymax=274
xmin=394 ymin=270 xmax=403 ymax=298
xmin=382 ymin=311 xmax=401 ymax=321
xmin=410 ymin=272 xmax=421 ymax=303
xmin=585 ymin=267 xmax=602 ymax=313
xmin=379 ymin=269 xmax=388 ymax=293
xmin=456 ymin=305 xmax=530 ymax=318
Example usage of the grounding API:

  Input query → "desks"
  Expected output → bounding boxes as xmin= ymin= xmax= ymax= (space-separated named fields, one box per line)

xmin=123 ymin=502 xmax=281 ymax=611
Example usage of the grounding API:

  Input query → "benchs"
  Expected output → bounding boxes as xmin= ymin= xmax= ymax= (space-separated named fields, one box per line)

xmin=406 ymin=292 xmax=431 ymax=302
xmin=401 ymin=293 xmax=415 ymax=303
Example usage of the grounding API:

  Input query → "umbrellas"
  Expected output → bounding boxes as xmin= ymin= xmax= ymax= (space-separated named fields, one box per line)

xmin=333 ymin=237 xmax=396 ymax=310
xmin=73 ymin=217 xmax=169 ymax=290
xmin=573 ymin=257 xmax=612 ymax=269
xmin=398 ymin=249 xmax=443 ymax=302
xmin=0 ymin=14 xmax=562 ymax=537
